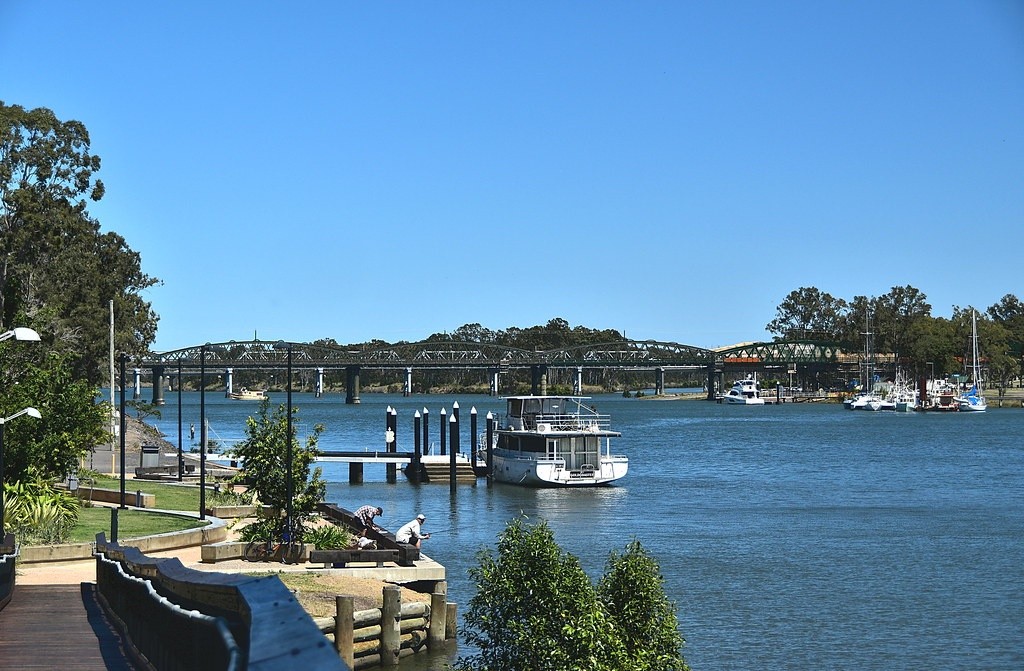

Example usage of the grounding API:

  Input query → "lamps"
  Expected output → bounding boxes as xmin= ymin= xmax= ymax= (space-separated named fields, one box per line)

xmin=552 ymin=399 xmax=559 ymax=409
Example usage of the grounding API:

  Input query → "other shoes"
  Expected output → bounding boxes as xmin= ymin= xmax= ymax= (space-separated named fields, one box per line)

xmin=419 ymin=555 xmax=425 ymax=560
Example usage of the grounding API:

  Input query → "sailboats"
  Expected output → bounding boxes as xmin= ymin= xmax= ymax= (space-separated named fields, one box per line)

xmin=842 ymin=308 xmax=988 ymax=411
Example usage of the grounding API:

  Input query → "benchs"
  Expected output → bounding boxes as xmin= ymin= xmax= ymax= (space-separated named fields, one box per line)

xmin=309 ymin=503 xmax=420 ymax=569
xmin=135 ymin=465 xmax=195 ymax=475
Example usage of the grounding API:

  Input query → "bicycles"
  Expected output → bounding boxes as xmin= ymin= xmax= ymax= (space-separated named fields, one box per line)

xmin=244 ymin=524 xmax=304 ymax=565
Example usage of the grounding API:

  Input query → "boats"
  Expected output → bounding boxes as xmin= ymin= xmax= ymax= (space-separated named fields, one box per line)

xmin=227 ymin=386 xmax=269 ymax=401
xmin=723 ymin=380 xmax=765 ymax=405
xmin=476 ymin=393 xmax=629 ymax=489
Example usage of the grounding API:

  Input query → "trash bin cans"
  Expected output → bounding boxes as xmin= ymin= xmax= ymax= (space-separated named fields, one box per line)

xmin=139 ymin=446 xmax=161 ymax=468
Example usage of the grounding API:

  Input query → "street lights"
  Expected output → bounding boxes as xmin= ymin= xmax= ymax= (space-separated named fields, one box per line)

xmin=273 ymin=341 xmax=293 ymax=543
xmin=199 ymin=344 xmax=218 ymax=521
xmin=118 ymin=352 xmax=131 ymax=511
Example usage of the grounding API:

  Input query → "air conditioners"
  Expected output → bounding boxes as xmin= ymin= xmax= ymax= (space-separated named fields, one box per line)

xmin=536 ymin=423 xmax=551 ymax=433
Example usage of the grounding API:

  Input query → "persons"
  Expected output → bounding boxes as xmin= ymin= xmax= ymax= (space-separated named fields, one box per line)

xmin=354 ymin=505 xmax=383 ymax=537
xmin=396 ymin=513 xmax=429 ymax=560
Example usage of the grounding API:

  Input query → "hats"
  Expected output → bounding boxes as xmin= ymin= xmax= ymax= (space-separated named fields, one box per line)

xmin=377 ymin=507 xmax=383 ymax=517
xmin=417 ymin=514 xmax=426 ymax=519
